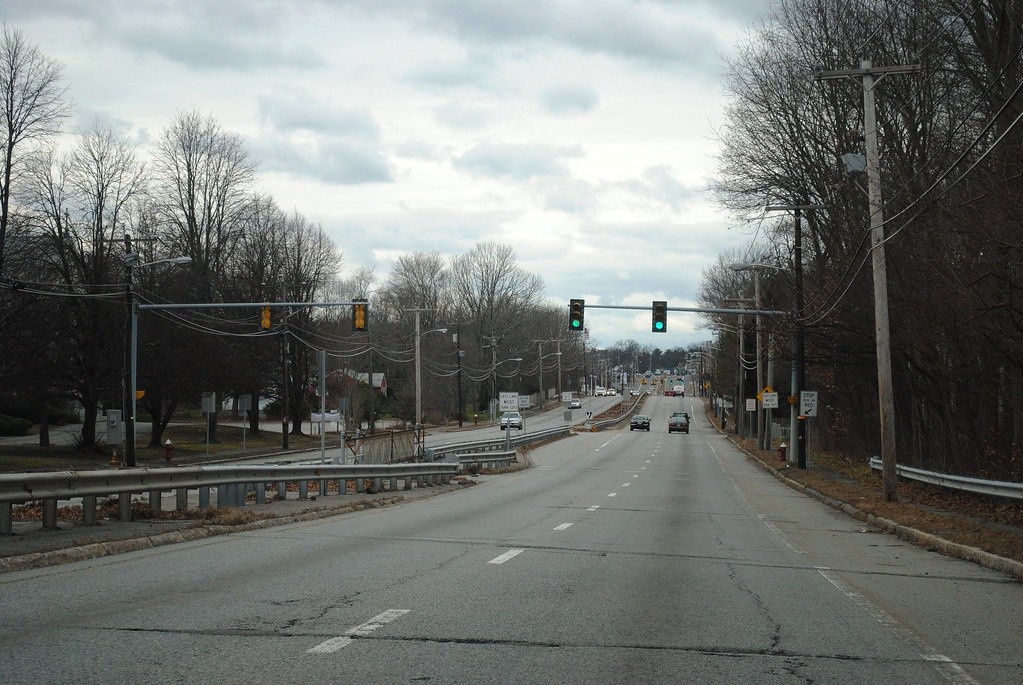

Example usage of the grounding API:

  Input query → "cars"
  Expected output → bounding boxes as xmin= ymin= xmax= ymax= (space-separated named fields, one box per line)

xmin=629 ymin=415 xmax=651 ymax=431
xmin=632 ymin=379 xmax=657 ymax=395
xmin=594 ymin=387 xmax=606 ymax=397
xmin=665 ymin=377 xmax=685 ymax=397
xmin=606 ymin=389 xmax=616 ymax=396
xmin=668 ymin=412 xmax=690 ymax=433
xmin=569 ymin=398 xmax=581 ymax=407
xmin=499 ymin=411 xmax=523 ymax=431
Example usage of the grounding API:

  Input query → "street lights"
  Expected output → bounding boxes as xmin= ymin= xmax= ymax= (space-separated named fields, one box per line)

xmin=687 ymin=323 xmax=742 ymax=434
xmin=125 ymin=255 xmax=191 ymax=459
xmin=539 ymin=352 xmax=562 ymax=410
xmin=416 ymin=329 xmax=447 ymax=426
xmin=728 ymin=262 xmax=799 ymax=463
xmin=493 ymin=358 xmax=522 ymax=423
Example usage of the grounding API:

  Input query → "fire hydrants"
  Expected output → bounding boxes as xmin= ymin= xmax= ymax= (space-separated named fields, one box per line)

xmin=164 ymin=439 xmax=173 ymax=462
xmin=777 ymin=443 xmax=788 ymax=463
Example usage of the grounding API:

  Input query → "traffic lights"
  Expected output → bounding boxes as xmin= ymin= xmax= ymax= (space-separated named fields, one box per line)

xmin=569 ymin=299 xmax=585 ymax=331
xmin=651 ymin=301 xmax=666 ymax=333
